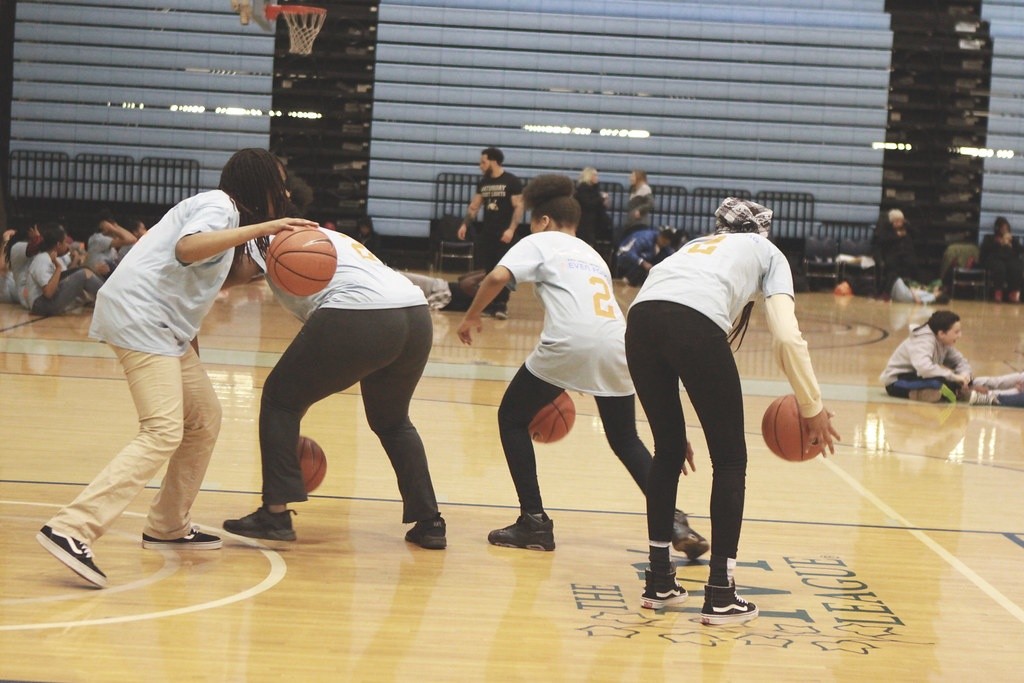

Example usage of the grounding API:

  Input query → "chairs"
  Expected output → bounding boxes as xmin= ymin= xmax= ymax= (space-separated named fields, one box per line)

xmin=432 ymin=213 xmax=1024 ymax=300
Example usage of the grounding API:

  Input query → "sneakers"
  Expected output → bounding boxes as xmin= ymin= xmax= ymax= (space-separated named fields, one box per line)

xmin=968 ymin=389 xmax=990 ymax=406
xmin=36 ymin=525 xmax=108 ymax=587
xmin=142 ymin=528 xmax=223 ymax=549
xmin=406 ymin=512 xmax=448 ymax=548
xmin=640 ymin=566 xmax=688 ymax=609
xmin=488 ymin=512 xmax=556 ymax=551
xmin=671 ymin=511 xmax=710 ymax=559
xmin=224 ymin=505 xmax=297 ymax=540
xmin=700 ymin=581 xmax=759 ymax=624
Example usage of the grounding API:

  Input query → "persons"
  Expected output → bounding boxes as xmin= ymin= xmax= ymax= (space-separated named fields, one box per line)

xmin=628 ymin=171 xmax=653 ymax=226
xmin=613 ymin=226 xmax=690 ymax=283
xmin=219 ymin=176 xmax=447 ymax=552
xmin=875 ymin=210 xmax=919 ymax=279
xmin=400 ymin=271 xmax=508 ymax=314
xmin=457 ymin=176 xmax=708 ymax=559
xmin=0 ymin=209 xmax=147 ymax=316
xmin=882 ymin=310 xmax=1024 ymax=408
xmin=577 ymin=167 xmax=609 ymax=244
xmin=836 ymin=253 xmax=948 ymax=305
xmin=982 ymin=217 xmax=1024 ymax=304
xmin=36 ymin=149 xmax=320 ymax=588
xmin=459 ymin=149 xmax=524 ymax=277
xmin=626 ymin=196 xmax=839 ymax=625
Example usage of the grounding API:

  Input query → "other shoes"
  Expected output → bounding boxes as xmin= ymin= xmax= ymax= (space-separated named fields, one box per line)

xmin=909 ymin=389 xmax=942 ymax=401
xmin=495 ymin=310 xmax=508 ymax=318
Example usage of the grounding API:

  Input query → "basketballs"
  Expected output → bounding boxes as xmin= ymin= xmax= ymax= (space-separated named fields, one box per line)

xmin=528 ymin=391 xmax=576 ymax=443
xmin=265 ymin=226 xmax=337 ymax=298
xmin=761 ymin=394 xmax=830 ymax=463
xmin=292 ymin=437 xmax=327 ymax=493
